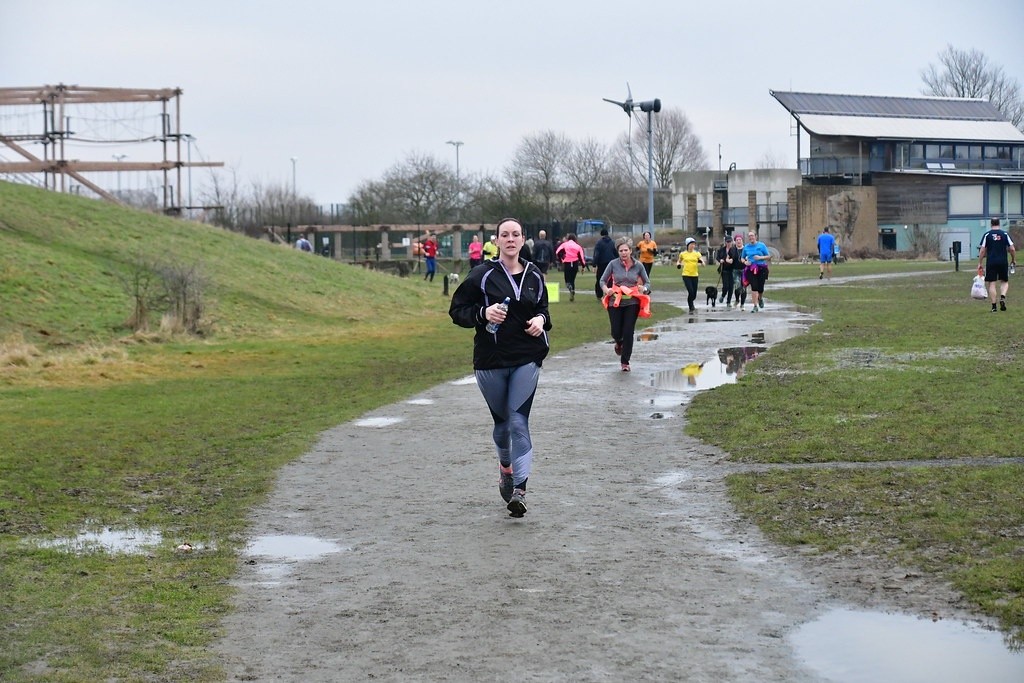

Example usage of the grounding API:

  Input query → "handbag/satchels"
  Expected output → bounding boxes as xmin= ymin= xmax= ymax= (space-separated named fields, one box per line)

xmin=971 ymin=269 xmax=988 ymax=300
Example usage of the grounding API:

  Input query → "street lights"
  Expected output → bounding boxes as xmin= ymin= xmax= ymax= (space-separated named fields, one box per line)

xmin=446 ymin=140 xmax=464 ymax=222
xmin=290 ymin=155 xmax=297 ymax=234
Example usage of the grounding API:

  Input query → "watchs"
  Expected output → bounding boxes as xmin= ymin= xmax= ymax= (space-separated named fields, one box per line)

xmin=643 ymin=287 xmax=647 ymax=293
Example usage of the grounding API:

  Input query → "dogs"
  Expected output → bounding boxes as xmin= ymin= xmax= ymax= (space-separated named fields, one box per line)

xmin=448 ymin=273 xmax=459 ymax=284
xmin=704 ymin=286 xmax=718 ymax=306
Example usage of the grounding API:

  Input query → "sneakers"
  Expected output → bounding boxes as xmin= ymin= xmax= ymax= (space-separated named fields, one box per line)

xmin=621 ymin=363 xmax=630 ymax=371
xmin=497 ymin=462 xmax=514 ymax=503
xmin=614 ymin=340 xmax=623 ymax=355
xmin=506 ymin=489 xmax=527 ymax=518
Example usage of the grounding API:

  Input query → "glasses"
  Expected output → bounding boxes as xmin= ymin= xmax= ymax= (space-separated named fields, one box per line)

xmin=726 ymin=240 xmax=733 ymax=243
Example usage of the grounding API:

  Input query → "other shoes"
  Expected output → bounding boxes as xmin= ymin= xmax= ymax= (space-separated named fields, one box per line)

xmin=758 ymin=297 xmax=764 ymax=308
xmin=734 ymin=301 xmax=739 ymax=306
xmin=689 ymin=308 xmax=695 ymax=314
xmin=751 ymin=306 xmax=758 ymax=313
xmin=567 ymin=291 xmax=576 ymax=301
xmin=1000 ymin=298 xmax=1006 ymax=311
xmin=740 ymin=304 xmax=745 ymax=311
xmin=719 ymin=297 xmax=724 ymax=303
xmin=819 ymin=271 xmax=824 ymax=279
xmin=727 ymin=303 xmax=731 ymax=310
xmin=992 ymin=308 xmax=997 ymax=312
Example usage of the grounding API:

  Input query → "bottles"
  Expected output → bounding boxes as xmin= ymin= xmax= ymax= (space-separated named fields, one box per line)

xmin=1010 ymin=262 xmax=1015 ymax=273
xmin=485 ymin=297 xmax=511 ymax=333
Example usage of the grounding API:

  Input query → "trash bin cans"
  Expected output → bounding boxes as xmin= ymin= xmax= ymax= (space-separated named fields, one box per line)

xmin=879 ymin=227 xmax=896 ymax=251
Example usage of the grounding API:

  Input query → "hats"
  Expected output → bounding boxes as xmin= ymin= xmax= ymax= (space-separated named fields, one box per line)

xmin=685 ymin=237 xmax=695 ymax=245
xmin=725 ymin=236 xmax=733 ymax=242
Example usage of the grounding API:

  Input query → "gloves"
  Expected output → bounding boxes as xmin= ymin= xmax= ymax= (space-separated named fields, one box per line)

xmin=677 ymin=265 xmax=681 ymax=269
xmin=698 ymin=260 xmax=702 ymax=263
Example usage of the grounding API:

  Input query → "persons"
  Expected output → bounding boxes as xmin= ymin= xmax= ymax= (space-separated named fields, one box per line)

xmin=677 ymin=238 xmax=705 ymax=310
xmin=818 ymin=227 xmax=834 ymax=280
xmin=469 ymin=235 xmax=483 ymax=269
xmin=636 ymin=232 xmax=657 ymax=295
xmin=519 ymin=230 xmax=567 ymax=281
xmin=556 ymin=233 xmax=585 ymax=301
xmin=716 ymin=230 xmax=770 ymax=313
xmin=592 ymin=230 xmax=619 ymax=300
xmin=600 ymin=237 xmax=651 ymax=370
xmin=421 ymin=233 xmax=438 ymax=282
xmin=483 ymin=235 xmax=497 ymax=260
xmin=680 ymin=329 xmax=768 ymax=388
xmin=449 ymin=218 xmax=552 ymax=518
xmin=296 ymin=234 xmax=313 ymax=253
xmin=978 ymin=218 xmax=1016 ymax=312
